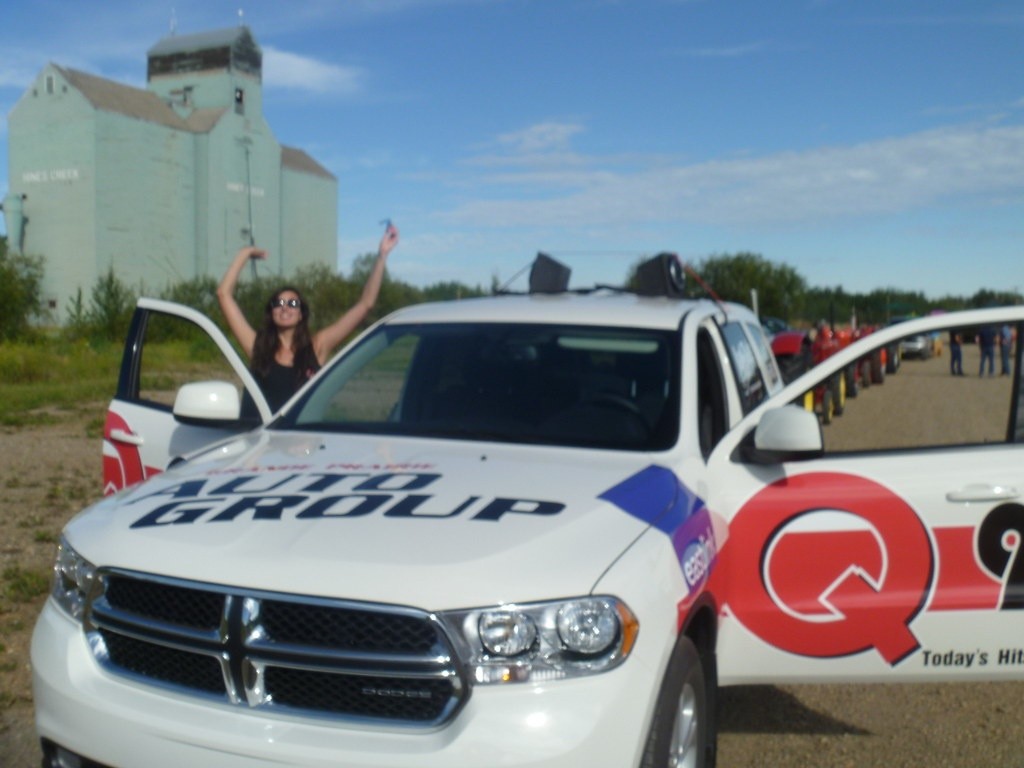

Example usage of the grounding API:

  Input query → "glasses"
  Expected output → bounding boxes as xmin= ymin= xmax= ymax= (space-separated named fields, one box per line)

xmin=271 ymin=298 xmax=300 ymax=308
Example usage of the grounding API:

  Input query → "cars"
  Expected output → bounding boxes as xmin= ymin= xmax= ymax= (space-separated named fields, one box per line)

xmin=25 ymin=289 xmax=1024 ymax=768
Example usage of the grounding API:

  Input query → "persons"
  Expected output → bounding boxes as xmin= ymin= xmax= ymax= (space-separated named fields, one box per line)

xmin=800 ymin=297 xmax=1022 ymax=428
xmin=214 ymin=218 xmax=401 ymax=425
